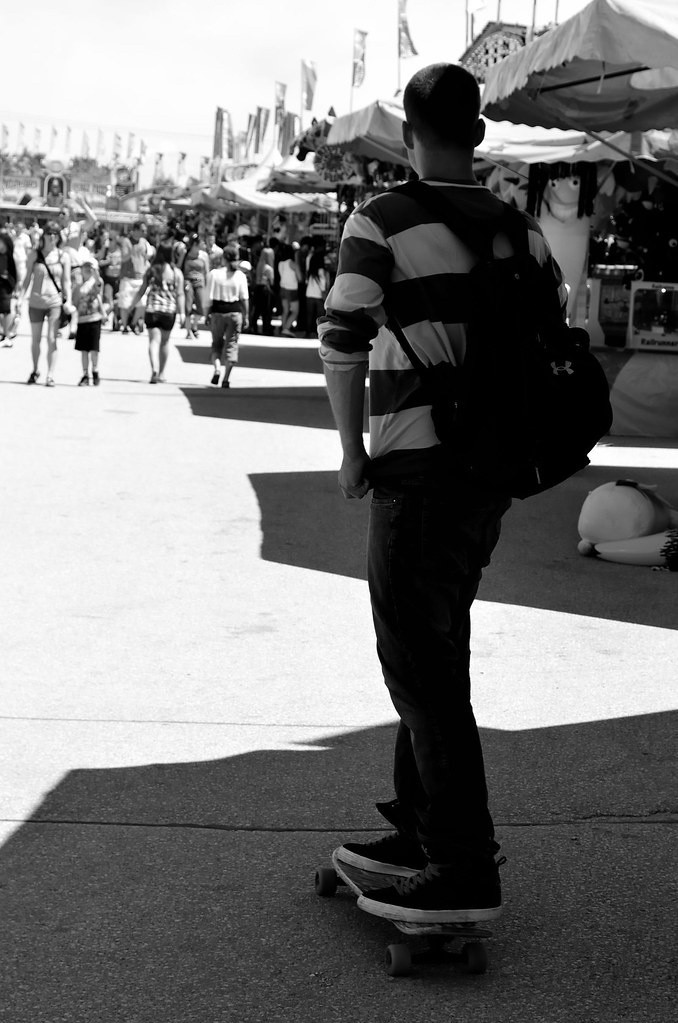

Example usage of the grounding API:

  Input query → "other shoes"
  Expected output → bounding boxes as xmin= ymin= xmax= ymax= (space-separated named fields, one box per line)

xmin=150 ymin=371 xmax=157 ymax=384
xmin=28 ymin=370 xmax=40 ymax=383
xmin=281 ymin=329 xmax=296 ymax=337
xmin=129 ymin=322 xmax=140 ymax=335
xmin=191 ymin=328 xmax=199 ymax=338
xmin=222 ymin=381 xmax=229 ymax=387
xmin=45 ymin=377 xmax=55 ymax=386
xmin=211 ymin=371 xmax=220 ymax=385
xmin=122 ymin=328 xmax=128 ymax=335
xmin=186 ymin=335 xmax=192 ymax=339
xmin=156 ymin=377 xmax=168 ymax=383
xmin=92 ymin=371 xmax=100 ymax=385
xmin=78 ymin=376 xmax=90 ymax=386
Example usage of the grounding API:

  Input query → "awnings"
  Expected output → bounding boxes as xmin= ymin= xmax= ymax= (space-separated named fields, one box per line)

xmin=190 ymin=174 xmax=271 ymax=241
xmin=260 ymin=106 xmax=370 ymax=191
xmin=327 ymin=81 xmax=678 ymax=176
xmin=216 ymin=140 xmax=358 ymax=215
xmin=288 ymin=115 xmax=405 ymax=175
xmin=480 ymin=0 xmax=678 ymax=186
xmin=164 ymin=195 xmax=197 ymax=211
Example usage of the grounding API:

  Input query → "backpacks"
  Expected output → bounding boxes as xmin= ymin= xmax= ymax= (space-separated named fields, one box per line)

xmin=369 ymin=180 xmax=613 ymax=499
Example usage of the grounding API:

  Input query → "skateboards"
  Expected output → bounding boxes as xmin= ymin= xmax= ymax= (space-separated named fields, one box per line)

xmin=314 ymin=845 xmax=495 ymax=976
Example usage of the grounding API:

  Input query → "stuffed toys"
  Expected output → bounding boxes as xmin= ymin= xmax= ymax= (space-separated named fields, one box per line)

xmin=578 ymin=478 xmax=678 ymax=554
xmin=523 ymin=162 xmax=597 ymax=318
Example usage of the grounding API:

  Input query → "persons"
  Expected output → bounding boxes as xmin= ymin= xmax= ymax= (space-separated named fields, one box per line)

xmin=317 ymin=63 xmax=568 ymax=921
xmin=72 ymin=260 xmax=108 ymax=386
xmin=204 ymin=244 xmax=249 ymax=387
xmin=17 ymin=221 xmax=74 ymax=387
xmin=128 ymin=240 xmax=187 ymax=382
xmin=0 ymin=194 xmax=337 ymax=351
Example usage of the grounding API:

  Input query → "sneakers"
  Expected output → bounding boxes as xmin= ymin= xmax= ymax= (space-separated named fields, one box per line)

xmin=338 ymin=832 xmax=429 ymax=877
xmin=357 ymin=840 xmax=507 ymax=923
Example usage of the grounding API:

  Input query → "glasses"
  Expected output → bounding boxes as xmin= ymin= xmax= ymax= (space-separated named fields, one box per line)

xmin=46 ymin=233 xmax=58 ymax=235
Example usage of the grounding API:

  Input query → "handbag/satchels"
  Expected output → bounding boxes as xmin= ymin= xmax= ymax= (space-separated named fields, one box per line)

xmin=306 ymin=276 xmax=322 ymax=299
xmin=56 ymin=298 xmax=72 ymax=329
